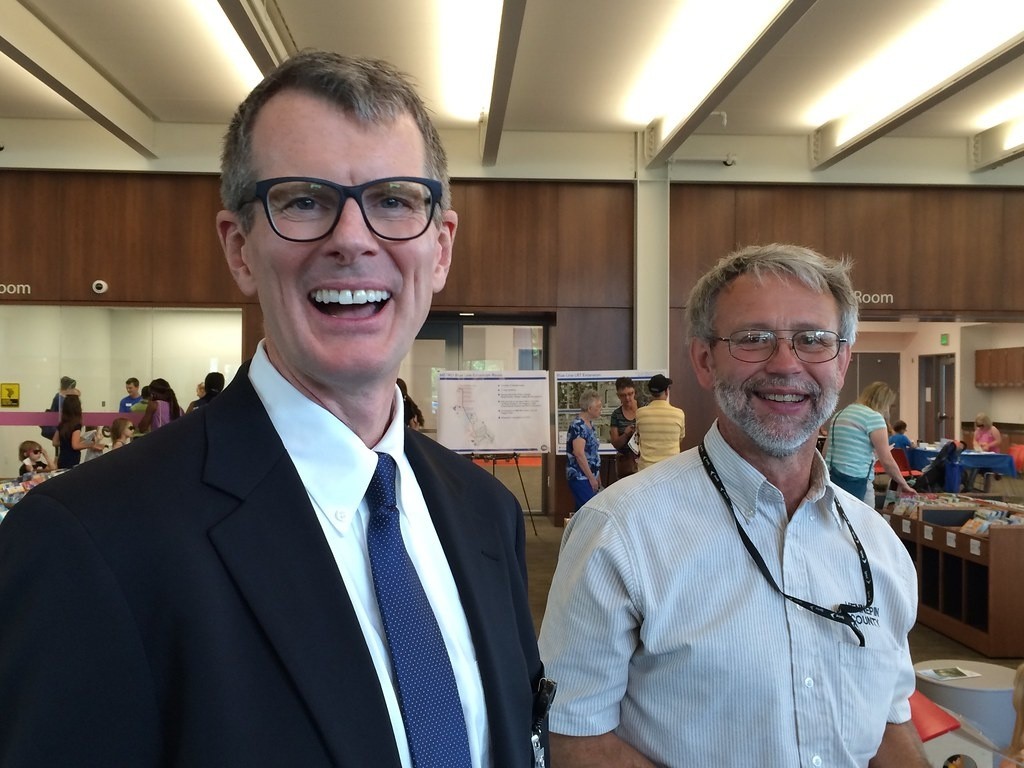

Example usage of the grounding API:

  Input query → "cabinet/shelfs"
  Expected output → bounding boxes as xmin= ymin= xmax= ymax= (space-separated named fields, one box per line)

xmin=973 ymin=346 xmax=1024 ymax=387
xmin=962 ymin=428 xmax=1024 ymax=477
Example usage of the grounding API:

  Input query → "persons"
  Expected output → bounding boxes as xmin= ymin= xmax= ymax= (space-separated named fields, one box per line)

xmin=537 ymin=242 xmax=936 ymax=768
xmin=611 ymin=374 xmax=686 ymax=479
xmin=820 ymin=380 xmax=1003 ymax=511
xmin=396 ymin=379 xmax=424 ymax=431
xmin=0 ymin=51 xmax=556 ymax=768
xmin=566 ymin=390 xmax=603 ymax=514
xmin=18 ymin=372 xmax=226 ymax=479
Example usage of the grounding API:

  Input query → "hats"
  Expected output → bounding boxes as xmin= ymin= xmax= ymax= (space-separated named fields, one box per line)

xmin=648 ymin=374 xmax=673 ymax=393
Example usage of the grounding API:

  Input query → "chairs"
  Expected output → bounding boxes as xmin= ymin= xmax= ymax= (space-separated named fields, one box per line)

xmin=875 ymin=449 xmax=1019 ymax=501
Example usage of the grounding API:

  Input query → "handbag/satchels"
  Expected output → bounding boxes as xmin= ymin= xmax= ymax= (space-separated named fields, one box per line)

xmin=828 ymin=467 xmax=868 ymax=500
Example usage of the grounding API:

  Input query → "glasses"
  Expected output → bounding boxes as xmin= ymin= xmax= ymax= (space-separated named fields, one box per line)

xmin=129 ymin=426 xmax=134 ymax=430
xmin=239 ymin=176 xmax=444 ymax=243
xmin=33 ymin=449 xmax=42 ymax=454
xmin=707 ymin=329 xmax=849 ymax=364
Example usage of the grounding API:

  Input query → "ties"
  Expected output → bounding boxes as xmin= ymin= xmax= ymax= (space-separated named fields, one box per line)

xmin=362 ymin=451 xmax=477 ymax=768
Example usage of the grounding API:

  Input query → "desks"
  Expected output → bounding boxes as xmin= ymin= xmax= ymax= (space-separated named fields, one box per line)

xmin=907 ymin=444 xmax=1024 ymax=497
xmin=874 ymin=496 xmax=1023 ymax=656
xmin=912 ymin=660 xmax=1018 ymax=752
